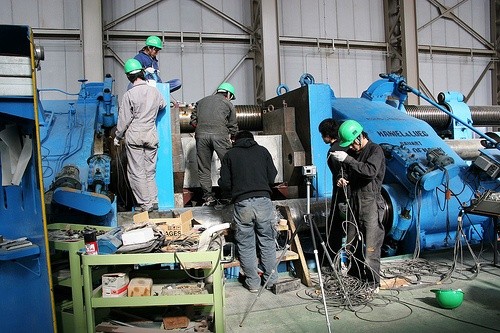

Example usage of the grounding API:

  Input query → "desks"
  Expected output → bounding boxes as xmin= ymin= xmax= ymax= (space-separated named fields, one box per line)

xmin=464 ymin=206 xmax=500 ymax=269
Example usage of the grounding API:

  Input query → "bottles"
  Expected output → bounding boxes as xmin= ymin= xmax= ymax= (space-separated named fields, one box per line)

xmin=83 ymin=231 xmax=98 ymax=256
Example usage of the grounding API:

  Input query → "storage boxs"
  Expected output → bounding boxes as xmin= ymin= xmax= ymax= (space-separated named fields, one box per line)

xmin=133 ymin=210 xmax=193 ymax=240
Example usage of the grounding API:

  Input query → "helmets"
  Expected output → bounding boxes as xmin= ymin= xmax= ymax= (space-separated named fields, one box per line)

xmin=217 ymin=83 xmax=236 ymax=100
xmin=145 ymin=35 xmax=162 ymax=49
xmin=338 ymin=120 xmax=363 ymax=148
xmin=124 ymin=59 xmax=142 ymax=74
xmin=430 ymin=289 xmax=465 ymax=308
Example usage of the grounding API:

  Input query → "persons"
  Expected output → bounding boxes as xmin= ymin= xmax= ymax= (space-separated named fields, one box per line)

xmin=113 ymin=58 xmax=168 ymax=213
xmin=318 ymin=118 xmax=387 ymax=288
xmin=126 ymin=35 xmax=182 ymax=94
xmin=189 ymin=82 xmax=236 ymax=207
xmin=217 ymin=131 xmax=278 ymax=293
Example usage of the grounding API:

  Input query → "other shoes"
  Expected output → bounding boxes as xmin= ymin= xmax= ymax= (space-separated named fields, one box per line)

xmin=364 ymin=287 xmax=380 ymax=294
xmin=249 ymin=288 xmax=258 ymax=293
xmin=266 ymin=285 xmax=273 ymax=289
xmin=152 ymin=203 xmax=158 ymax=210
xmin=203 ymin=196 xmax=215 ymax=205
xmin=142 ymin=207 xmax=152 ymax=213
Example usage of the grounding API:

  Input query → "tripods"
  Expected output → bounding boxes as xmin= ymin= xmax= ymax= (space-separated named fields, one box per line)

xmin=238 ymin=180 xmax=355 ymax=333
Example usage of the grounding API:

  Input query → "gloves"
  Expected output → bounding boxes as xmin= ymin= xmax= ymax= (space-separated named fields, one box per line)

xmin=114 ymin=138 xmax=120 ymax=146
xmin=329 ymin=151 xmax=348 ymax=162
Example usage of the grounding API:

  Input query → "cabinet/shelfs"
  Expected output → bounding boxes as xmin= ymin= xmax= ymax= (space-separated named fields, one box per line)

xmin=0 ymin=24 xmax=57 ymax=333
xmin=46 ymin=223 xmax=115 ymax=333
xmin=81 ymin=241 xmax=227 ymax=333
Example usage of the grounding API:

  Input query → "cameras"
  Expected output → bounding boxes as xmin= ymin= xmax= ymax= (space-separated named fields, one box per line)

xmin=302 ymin=166 xmax=316 ymax=176
